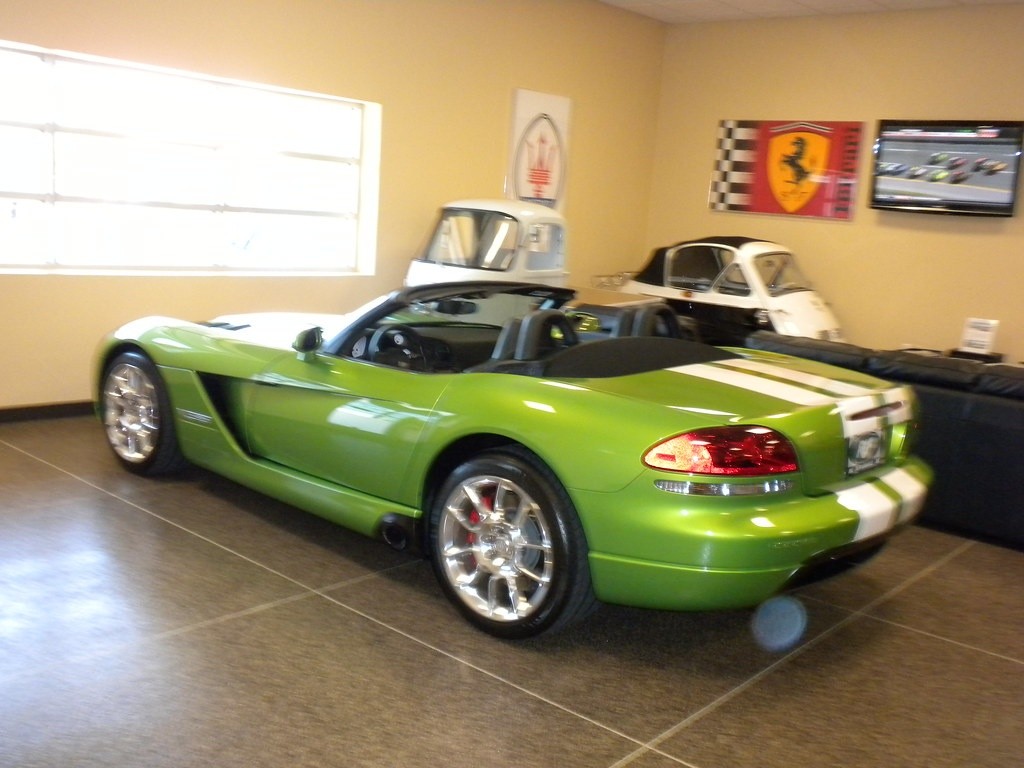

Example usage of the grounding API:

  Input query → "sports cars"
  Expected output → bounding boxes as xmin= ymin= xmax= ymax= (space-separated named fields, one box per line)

xmin=93 ymin=274 xmax=940 ymax=644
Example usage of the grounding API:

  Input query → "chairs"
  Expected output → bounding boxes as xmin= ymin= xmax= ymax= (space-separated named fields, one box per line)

xmin=610 ymin=304 xmax=684 ymax=341
xmin=452 ymin=307 xmax=578 ymax=374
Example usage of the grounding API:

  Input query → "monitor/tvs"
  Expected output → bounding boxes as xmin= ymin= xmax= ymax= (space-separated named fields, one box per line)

xmin=868 ymin=121 xmax=1024 ymax=217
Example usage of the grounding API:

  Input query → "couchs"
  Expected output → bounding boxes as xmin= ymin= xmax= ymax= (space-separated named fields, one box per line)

xmin=744 ymin=330 xmax=1024 ymax=553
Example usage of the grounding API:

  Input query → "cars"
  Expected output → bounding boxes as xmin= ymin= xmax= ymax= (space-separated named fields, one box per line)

xmin=406 ymin=202 xmax=566 ymax=289
xmin=624 ymin=234 xmax=836 ymax=341
